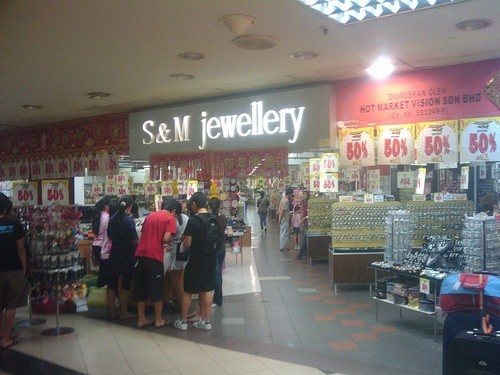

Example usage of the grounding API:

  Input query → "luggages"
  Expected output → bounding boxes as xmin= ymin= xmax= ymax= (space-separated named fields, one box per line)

xmin=443 ymin=310 xmax=500 ymax=375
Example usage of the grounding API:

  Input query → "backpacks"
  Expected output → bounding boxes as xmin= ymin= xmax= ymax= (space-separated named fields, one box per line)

xmin=193 ymin=213 xmax=226 ymax=259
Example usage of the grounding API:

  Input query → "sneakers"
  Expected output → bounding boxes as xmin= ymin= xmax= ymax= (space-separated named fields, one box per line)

xmin=186 ymin=312 xmax=202 ymax=322
xmin=173 ymin=315 xmax=188 ymax=331
xmin=193 ymin=319 xmax=212 ymax=331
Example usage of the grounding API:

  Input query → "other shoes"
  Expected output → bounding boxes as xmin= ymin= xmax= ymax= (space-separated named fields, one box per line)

xmin=110 ymin=312 xmax=118 ymax=319
xmin=280 ymin=247 xmax=291 ymax=251
xmin=259 ymin=230 xmax=264 ymax=232
xmin=264 ymin=226 xmax=268 ymax=232
xmin=119 ymin=311 xmax=137 ymax=320
xmin=294 ymin=245 xmax=301 ymax=250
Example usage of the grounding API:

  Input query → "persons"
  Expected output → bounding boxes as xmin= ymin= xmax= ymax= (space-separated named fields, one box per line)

xmin=174 ymin=192 xmax=218 ymax=331
xmin=0 ymin=193 xmax=27 ymax=350
xmin=292 ymin=207 xmax=303 ymax=250
xmin=107 ymin=195 xmax=139 ymax=320
xmin=476 ymin=193 xmax=500 ymax=220
xmin=135 ymin=197 xmax=182 ymax=328
xmin=256 ymin=191 xmax=270 ymax=232
xmin=95 ymin=195 xmax=120 ymax=308
xmin=277 ymin=189 xmax=294 ymax=251
xmin=170 ymin=197 xmax=226 ymax=323
xmin=296 ymin=191 xmax=309 ymax=259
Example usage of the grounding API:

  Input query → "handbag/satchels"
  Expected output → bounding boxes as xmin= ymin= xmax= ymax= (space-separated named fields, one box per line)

xmin=176 ymin=241 xmax=188 ymax=261
xmin=439 ymin=272 xmax=500 ymax=317
xmin=256 ymin=207 xmax=258 ymax=214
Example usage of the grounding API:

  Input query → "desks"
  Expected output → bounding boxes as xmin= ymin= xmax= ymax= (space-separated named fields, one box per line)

xmin=224 ymin=232 xmax=244 ymax=264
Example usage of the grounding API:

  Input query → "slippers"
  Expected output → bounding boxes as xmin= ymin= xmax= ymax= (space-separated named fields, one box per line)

xmin=154 ymin=319 xmax=171 ymax=328
xmin=0 ymin=334 xmax=18 ymax=351
xmin=137 ymin=319 xmax=154 ymax=328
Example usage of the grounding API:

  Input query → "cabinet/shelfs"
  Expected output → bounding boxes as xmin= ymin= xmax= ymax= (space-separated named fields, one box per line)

xmin=462 ymin=214 xmax=500 ymax=274
xmin=327 ymin=202 xmax=476 ymax=295
xmin=24 ymin=204 xmax=88 ymax=335
xmin=383 ymin=209 xmax=412 ymax=265
xmin=306 ymin=198 xmax=340 ymax=268
xmin=365 ymin=266 xmax=453 ymax=341
